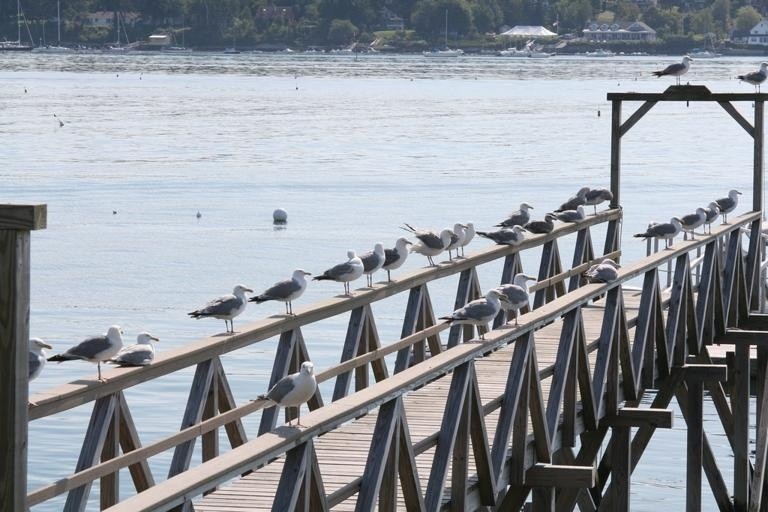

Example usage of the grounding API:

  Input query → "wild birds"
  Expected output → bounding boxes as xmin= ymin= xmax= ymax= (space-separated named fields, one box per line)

xmin=46 ymin=326 xmax=159 ymax=384
xmin=311 ymin=218 xmax=474 ymax=299
xmin=247 ymin=268 xmax=312 ymax=317
xmin=650 ymin=54 xmax=694 ymax=85
xmin=733 ymin=60 xmax=768 ymax=93
xmin=633 ymin=187 xmax=743 ymax=251
xmin=189 ymin=284 xmax=252 ymax=334
xmin=580 ymin=258 xmax=622 ymax=284
xmin=248 ymin=358 xmax=318 ymax=430
xmin=439 ymin=271 xmax=537 ymax=341
xmin=28 ymin=336 xmax=52 ymax=408
xmin=477 ymin=185 xmax=613 ymax=246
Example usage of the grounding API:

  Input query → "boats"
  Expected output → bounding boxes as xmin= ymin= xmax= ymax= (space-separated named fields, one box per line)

xmin=422 ymin=47 xmax=463 ymax=57
xmin=496 ymin=40 xmax=550 ymax=58
xmin=688 ymin=51 xmax=722 ymax=59
xmin=586 ymin=48 xmax=615 ymax=56
xmin=224 ymin=48 xmax=240 ymax=54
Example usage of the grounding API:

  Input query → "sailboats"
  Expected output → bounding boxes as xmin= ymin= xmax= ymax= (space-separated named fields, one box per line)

xmin=0 ymin=0 xmax=192 ymax=55
